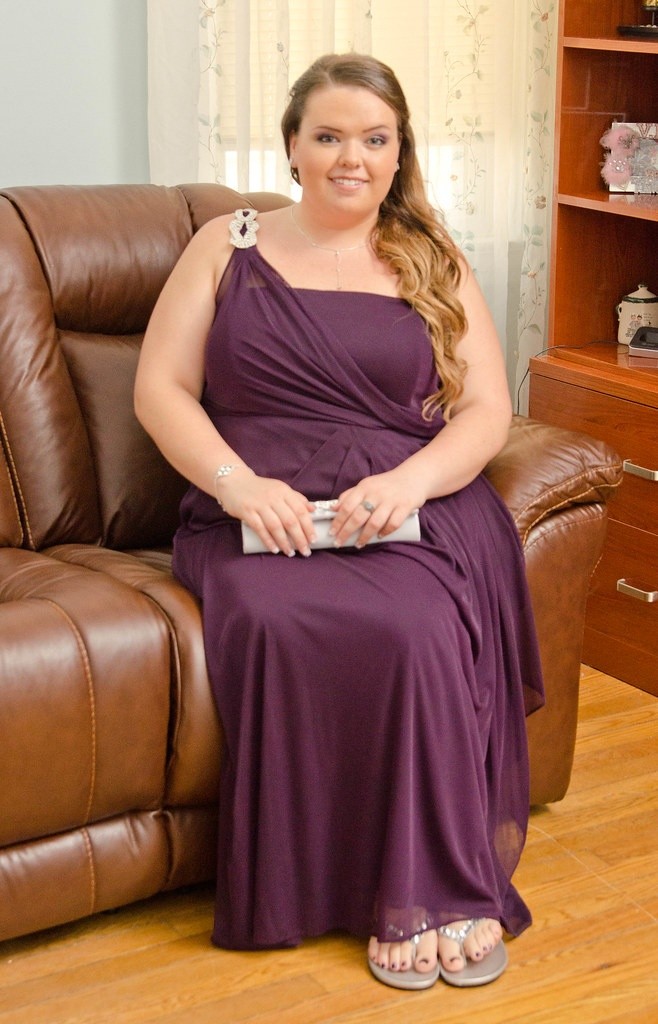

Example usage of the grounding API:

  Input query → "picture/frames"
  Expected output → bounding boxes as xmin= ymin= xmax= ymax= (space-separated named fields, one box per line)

xmin=609 ymin=122 xmax=658 ymax=195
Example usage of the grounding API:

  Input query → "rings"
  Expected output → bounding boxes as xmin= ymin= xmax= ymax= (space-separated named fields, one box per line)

xmin=363 ymin=500 xmax=375 ymax=513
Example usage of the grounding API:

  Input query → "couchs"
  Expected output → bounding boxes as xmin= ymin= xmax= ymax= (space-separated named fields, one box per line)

xmin=0 ymin=181 xmax=622 ymax=942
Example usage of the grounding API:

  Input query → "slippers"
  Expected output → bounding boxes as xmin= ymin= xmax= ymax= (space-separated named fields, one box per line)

xmin=434 ymin=909 xmax=508 ymax=986
xmin=368 ymin=899 xmax=439 ymax=991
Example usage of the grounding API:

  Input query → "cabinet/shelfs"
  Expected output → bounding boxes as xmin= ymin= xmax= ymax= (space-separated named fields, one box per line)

xmin=526 ymin=1 xmax=658 ymax=698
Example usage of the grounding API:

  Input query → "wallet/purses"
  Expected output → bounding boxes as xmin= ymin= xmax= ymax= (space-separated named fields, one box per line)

xmin=241 ymin=499 xmax=423 ymax=555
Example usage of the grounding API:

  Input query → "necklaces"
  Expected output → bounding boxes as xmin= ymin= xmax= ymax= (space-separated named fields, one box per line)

xmin=289 ymin=203 xmax=371 ymax=287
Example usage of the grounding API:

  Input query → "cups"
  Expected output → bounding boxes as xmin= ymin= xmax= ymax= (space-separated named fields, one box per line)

xmin=615 ymin=284 xmax=657 ymax=346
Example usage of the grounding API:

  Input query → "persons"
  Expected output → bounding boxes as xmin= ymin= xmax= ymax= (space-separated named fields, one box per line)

xmin=133 ymin=54 xmax=544 ymax=991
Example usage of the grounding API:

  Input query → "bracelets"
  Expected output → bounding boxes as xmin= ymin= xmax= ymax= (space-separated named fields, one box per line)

xmin=213 ymin=461 xmax=254 ymax=510
